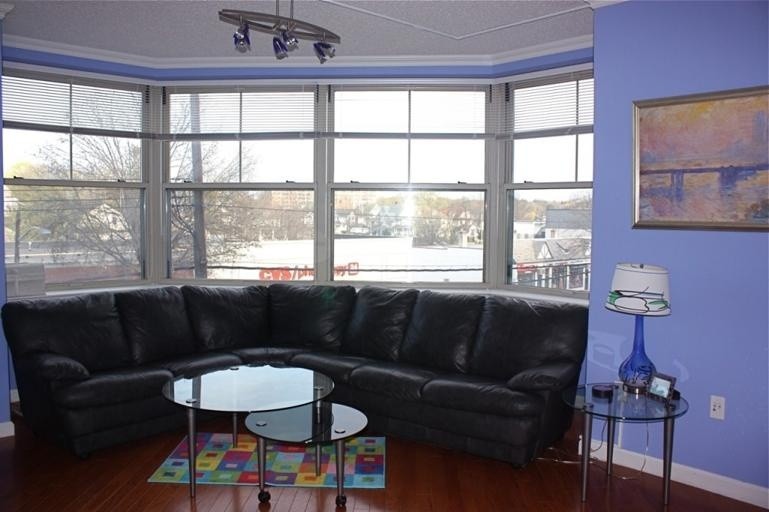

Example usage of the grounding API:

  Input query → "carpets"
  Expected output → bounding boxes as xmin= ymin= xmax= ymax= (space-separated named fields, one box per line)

xmin=148 ymin=433 xmax=386 ymax=490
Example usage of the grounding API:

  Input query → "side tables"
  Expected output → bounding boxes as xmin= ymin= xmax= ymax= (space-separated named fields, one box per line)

xmin=561 ymin=379 xmax=690 ymax=505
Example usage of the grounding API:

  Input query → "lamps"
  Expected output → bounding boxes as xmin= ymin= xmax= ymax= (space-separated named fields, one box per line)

xmin=602 ymin=262 xmax=674 ymax=396
xmin=218 ymin=0 xmax=342 ymax=64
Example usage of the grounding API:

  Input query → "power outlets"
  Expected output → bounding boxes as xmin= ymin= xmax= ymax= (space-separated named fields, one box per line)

xmin=709 ymin=395 xmax=726 ymax=420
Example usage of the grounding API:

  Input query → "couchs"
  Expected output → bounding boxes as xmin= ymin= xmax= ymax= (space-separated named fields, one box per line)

xmin=1 ymin=280 xmax=591 ymax=468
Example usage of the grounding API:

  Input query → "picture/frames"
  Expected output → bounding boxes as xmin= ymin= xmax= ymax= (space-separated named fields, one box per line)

xmin=632 ymin=84 xmax=769 ymax=230
